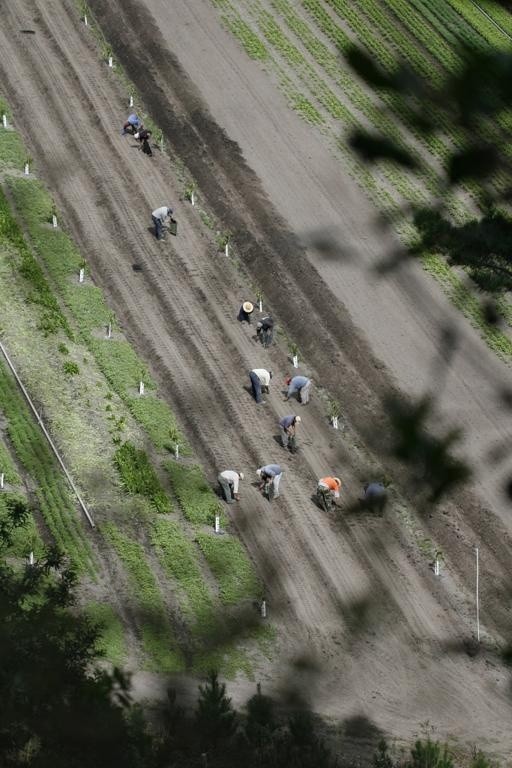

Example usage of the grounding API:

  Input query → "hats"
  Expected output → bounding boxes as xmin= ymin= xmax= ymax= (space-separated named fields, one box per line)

xmin=257 ymin=322 xmax=262 ymax=330
xmin=270 ymin=371 xmax=274 ymax=379
xmin=286 ymin=376 xmax=291 ymax=385
xmin=126 ymin=125 xmax=133 ymax=134
xmin=167 ymin=208 xmax=173 ymax=217
xmin=243 ymin=301 xmax=253 ymax=313
xmin=295 ymin=416 xmax=302 ymax=426
xmin=334 ymin=477 xmax=341 ymax=488
xmin=134 ymin=132 xmax=139 ymax=140
xmin=256 ymin=468 xmax=261 ymax=479
xmin=240 ymin=472 xmax=245 ymax=480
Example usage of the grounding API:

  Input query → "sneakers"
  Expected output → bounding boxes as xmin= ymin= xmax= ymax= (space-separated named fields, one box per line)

xmin=227 ymin=499 xmax=236 ymax=504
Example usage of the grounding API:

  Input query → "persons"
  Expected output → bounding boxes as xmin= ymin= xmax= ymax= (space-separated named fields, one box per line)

xmin=256 ymin=463 xmax=284 ymax=499
xmin=282 ymin=376 xmax=312 ymax=405
xmin=364 ymin=483 xmax=389 ymax=517
xmin=316 ymin=475 xmax=340 ymax=512
xmin=276 ymin=415 xmax=301 ymax=450
xmin=150 ymin=205 xmax=173 ymax=241
xmin=237 ymin=300 xmax=254 ymax=325
xmin=249 ymin=369 xmax=272 ymax=405
xmin=256 ymin=316 xmax=275 ymax=349
xmin=217 ymin=470 xmax=243 ymax=504
xmin=120 ymin=114 xmax=152 ymax=150
